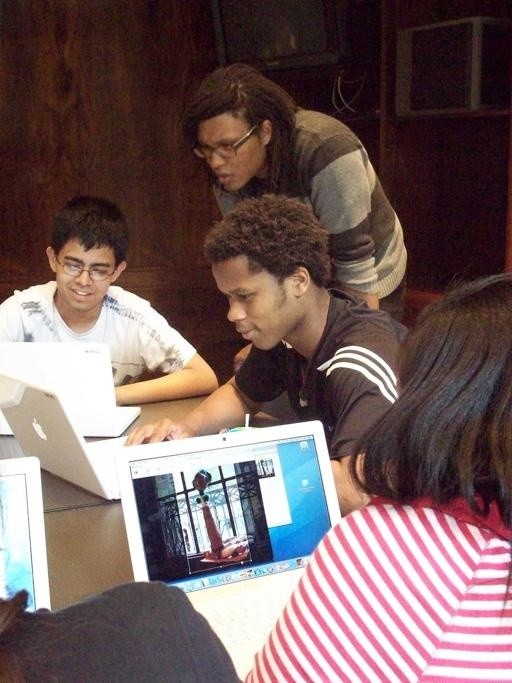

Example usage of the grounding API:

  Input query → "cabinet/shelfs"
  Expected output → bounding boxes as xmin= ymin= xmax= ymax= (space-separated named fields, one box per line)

xmin=282 ymin=0 xmax=512 ymax=303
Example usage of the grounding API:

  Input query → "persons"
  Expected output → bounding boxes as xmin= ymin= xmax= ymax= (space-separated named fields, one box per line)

xmin=0 ymin=580 xmax=244 ymax=683
xmin=122 ymin=194 xmax=411 ymax=520
xmin=245 ymin=273 xmax=512 ymax=683
xmin=1 ymin=193 xmax=218 ymax=409
xmin=185 ymin=62 xmax=415 ymax=374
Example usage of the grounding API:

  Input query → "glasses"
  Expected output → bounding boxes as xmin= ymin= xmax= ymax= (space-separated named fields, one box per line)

xmin=193 ymin=124 xmax=258 ymax=158
xmin=50 ymin=252 xmax=116 ymax=281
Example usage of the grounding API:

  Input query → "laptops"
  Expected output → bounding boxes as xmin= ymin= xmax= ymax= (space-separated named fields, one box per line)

xmin=0 ymin=340 xmax=141 ymax=437
xmin=116 ymin=420 xmax=341 ymax=683
xmin=0 ymin=373 xmax=144 ymax=503
xmin=0 ymin=456 xmax=53 ymax=613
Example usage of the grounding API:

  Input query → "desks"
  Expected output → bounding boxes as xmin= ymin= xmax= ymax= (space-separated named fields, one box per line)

xmin=40 ymin=500 xmax=136 ymax=613
xmin=36 ymin=382 xmax=296 ymax=510
